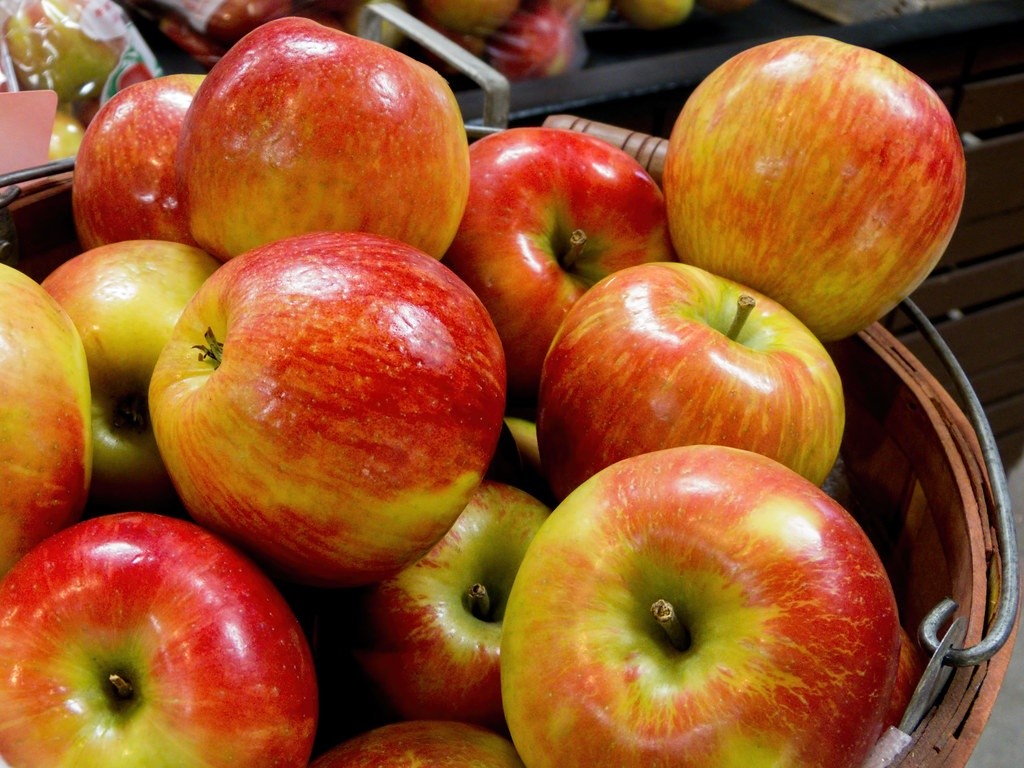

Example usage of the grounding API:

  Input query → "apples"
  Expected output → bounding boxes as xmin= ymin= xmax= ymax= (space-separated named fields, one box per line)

xmin=0 ymin=0 xmax=965 ymax=768
